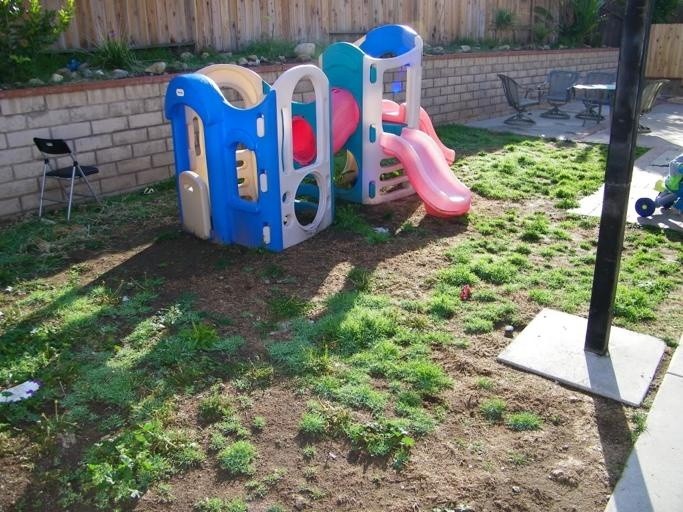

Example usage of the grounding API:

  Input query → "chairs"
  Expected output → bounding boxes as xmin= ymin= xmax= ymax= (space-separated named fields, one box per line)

xmin=32 ymin=137 xmax=101 ymax=224
xmin=496 ymin=70 xmax=669 ymax=134
